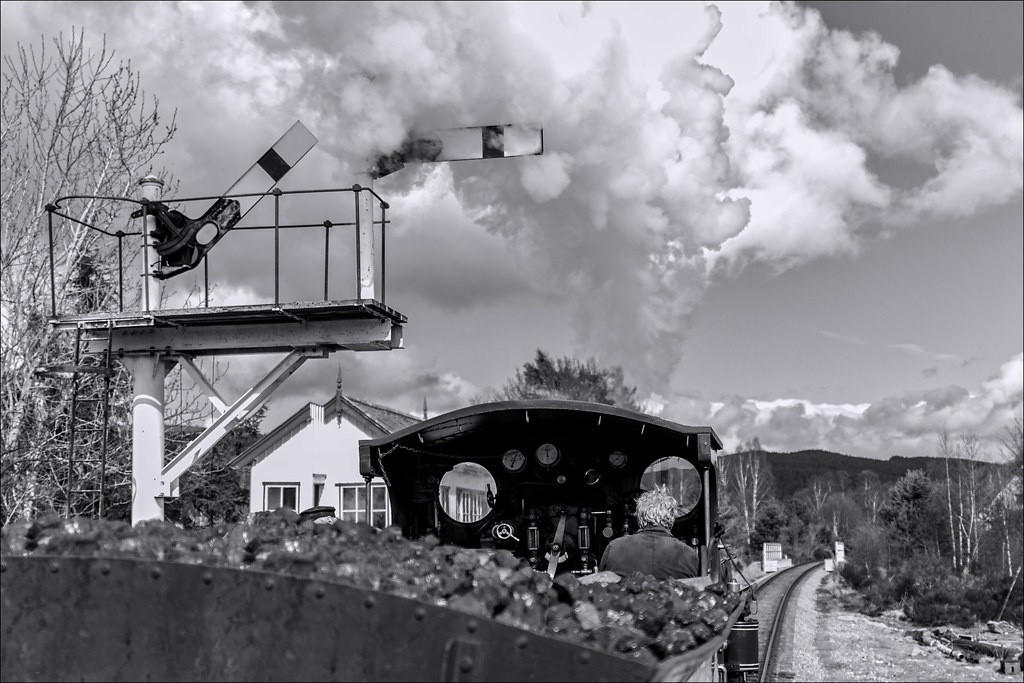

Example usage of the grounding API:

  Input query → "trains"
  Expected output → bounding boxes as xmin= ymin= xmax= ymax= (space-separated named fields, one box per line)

xmin=0 ymin=399 xmax=761 ymax=683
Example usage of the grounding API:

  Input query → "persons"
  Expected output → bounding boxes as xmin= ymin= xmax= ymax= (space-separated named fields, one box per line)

xmin=598 ymin=483 xmax=700 ymax=581
